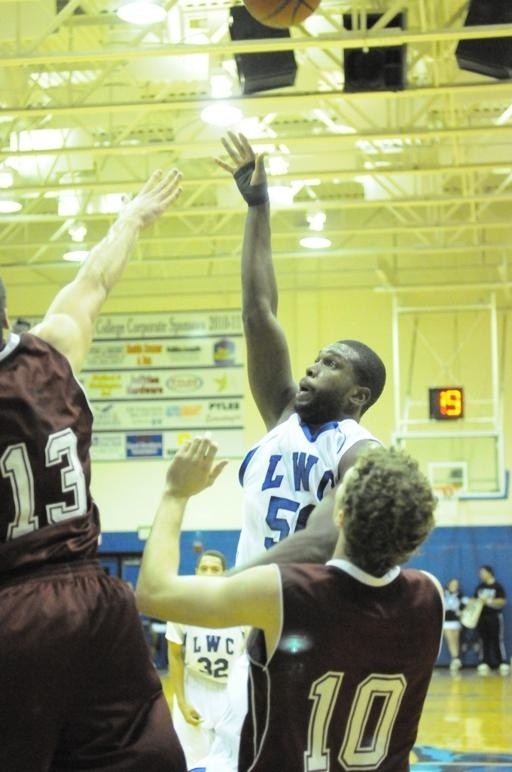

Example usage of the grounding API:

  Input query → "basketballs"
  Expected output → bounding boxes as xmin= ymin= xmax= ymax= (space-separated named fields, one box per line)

xmin=244 ymin=0 xmax=321 ymax=27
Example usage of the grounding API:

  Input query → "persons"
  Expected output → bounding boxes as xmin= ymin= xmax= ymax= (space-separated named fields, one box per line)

xmin=164 ymin=549 xmax=254 ymax=772
xmin=468 ymin=566 xmax=510 ymax=670
xmin=210 ymin=132 xmax=388 ymax=576
xmin=444 ymin=578 xmax=466 ymax=669
xmin=134 ymin=436 xmax=443 ymax=771
xmin=148 ymin=619 xmax=167 ymax=672
xmin=1 ymin=167 xmax=186 ymax=772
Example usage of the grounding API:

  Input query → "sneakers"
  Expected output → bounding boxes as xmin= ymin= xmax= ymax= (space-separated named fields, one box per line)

xmin=446 ymin=658 xmax=511 ymax=676
